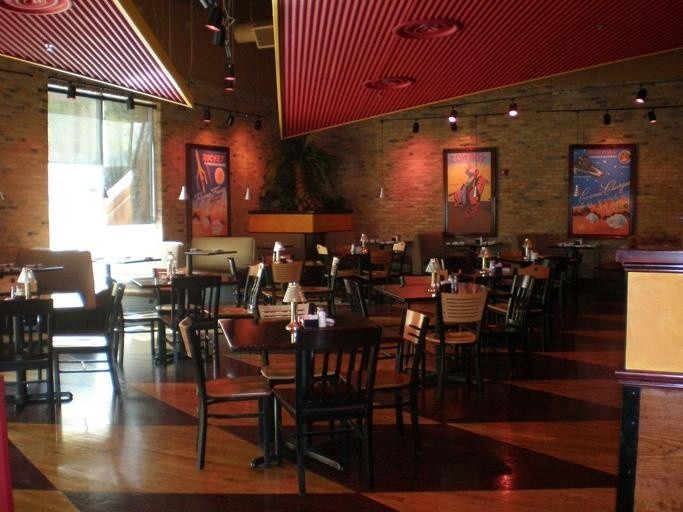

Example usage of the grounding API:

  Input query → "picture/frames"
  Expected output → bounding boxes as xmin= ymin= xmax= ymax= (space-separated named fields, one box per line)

xmin=185 ymin=143 xmax=231 ymax=238
xmin=442 ymin=145 xmax=498 ymax=237
xmin=567 ymin=143 xmax=639 ymax=238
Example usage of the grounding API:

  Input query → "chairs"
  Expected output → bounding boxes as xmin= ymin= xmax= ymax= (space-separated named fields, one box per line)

xmin=271 ymin=324 xmax=382 ymax=493
xmin=481 ymin=274 xmax=536 ymax=370
xmin=260 ymin=262 xmax=303 ymax=305
xmin=204 ymin=262 xmax=269 ymax=322
xmin=178 ymin=316 xmax=273 ymax=470
xmin=370 ymin=242 xmax=406 ymax=310
xmin=421 ymin=290 xmax=489 ymax=402
xmin=51 ymin=283 xmax=127 ymax=405
xmin=487 ymin=264 xmax=550 ymax=355
xmin=105 ymin=276 xmax=162 ymax=368
xmin=300 ymin=256 xmax=340 ymax=319
xmin=339 ymin=308 xmax=430 ymax=452
xmin=452 ymin=227 xmax=594 ymax=280
xmin=0 ymin=298 xmax=56 ymax=425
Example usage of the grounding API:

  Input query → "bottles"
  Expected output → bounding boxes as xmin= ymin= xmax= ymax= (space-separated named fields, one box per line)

xmin=360 ymin=234 xmax=366 ymax=250
xmin=496 ymin=252 xmax=502 ymax=277
xmin=439 ymin=274 xmax=446 ymax=292
xmin=319 ymin=310 xmax=327 ymax=331
xmin=489 ymin=260 xmax=495 ymax=277
xmin=10 ymin=285 xmax=23 ymax=300
xmin=448 ymin=275 xmax=457 ymax=293
xmin=350 ymin=243 xmax=356 ymax=255
xmin=167 ymin=252 xmax=177 ymax=282
xmin=523 ymin=240 xmax=534 ymax=262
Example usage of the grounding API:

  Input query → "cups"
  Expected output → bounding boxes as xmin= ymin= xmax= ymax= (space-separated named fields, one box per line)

xmin=449 ymin=275 xmax=457 ymax=293
xmin=318 ymin=311 xmax=327 ymax=328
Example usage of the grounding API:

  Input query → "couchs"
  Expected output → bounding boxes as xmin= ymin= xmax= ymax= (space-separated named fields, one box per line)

xmin=115 ymin=241 xmax=184 ymax=297
xmin=176 ymin=236 xmax=256 ymax=284
xmin=0 ymin=247 xmax=96 ymax=331
xmin=410 ymin=228 xmax=449 ymax=276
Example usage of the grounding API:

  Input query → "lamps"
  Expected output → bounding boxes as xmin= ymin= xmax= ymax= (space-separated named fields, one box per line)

xmin=254 ymin=116 xmax=261 ymax=131
xmin=412 ymin=118 xmax=419 ymax=134
xmin=448 ymin=106 xmax=458 ymax=123
xmin=125 ymin=93 xmax=134 ymax=110
xmin=223 ymin=63 xmax=236 ymax=81
xmin=379 ymin=119 xmax=384 ymax=199
xmin=647 ymin=108 xmax=657 ymax=124
xmin=205 ymin=1 xmax=224 ymax=32
xmin=635 ymin=85 xmax=648 ymax=104
xmin=177 ymin=105 xmax=190 ymax=200
xmin=508 ymin=98 xmax=518 ymax=117
xmin=243 ymin=112 xmax=251 ymax=200
xmin=66 ymin=80 xmax=76 ymax=99
xmin=202 ymin=107 xmax=210 ymax=123
xmin=223 ymin=79 xmax=235 ymax=91
xmin=226 ymin=112 xmax=234 ymax=127
xmin=602 ymin=110 xmax=611 ymax=125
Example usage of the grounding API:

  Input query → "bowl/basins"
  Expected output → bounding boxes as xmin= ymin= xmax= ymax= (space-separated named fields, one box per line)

xmin=326 ymin=317 xmax=335 ymax=327
